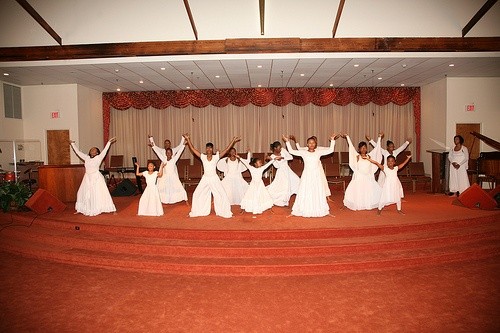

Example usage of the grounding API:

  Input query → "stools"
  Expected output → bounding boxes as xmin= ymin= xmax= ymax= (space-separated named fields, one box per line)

xmin=23 ymin=179 xmax=36 ymax=192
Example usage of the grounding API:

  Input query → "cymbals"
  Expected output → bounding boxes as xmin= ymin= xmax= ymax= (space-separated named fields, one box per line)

xmin=24 ymin=168 xmax=32 ymax=174
xmin=21 ymin=179 xmax=36 ymax=184
xmin=9 ymin=162 xmax=24 ymax=165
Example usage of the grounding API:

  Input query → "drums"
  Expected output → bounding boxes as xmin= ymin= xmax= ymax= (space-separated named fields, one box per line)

xmin=14 ymin=171 xmax=21 ymax=182
xmin=0 ymin=172 xmax=6 ymax=181
xmin=5 ymin=171 xmax=15 ymax=182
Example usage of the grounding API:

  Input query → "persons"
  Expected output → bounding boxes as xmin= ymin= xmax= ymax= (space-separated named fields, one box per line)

xmin=146 ymin=134 xmax=187 ymax=165
xmin=362 ymin=155 xmax=413 ymax=216
xmin=64 ymin=136 xmax=119 ymax=216
xmin=147 ymin=143 xmax=190 ymax=208
xmin=341 ymin=131 xmax=385 ymax=213
xmin=448 ymin=136 xmax=471 ymax=200
xmin=263 ymin=141 xmax=301 ymax=204
xmin=235 ymin=154 xmax=284 ymax=216
xmin=184 ymin=133 xmax=240 ymax=218
xmin=134 ymin=162 xmax=167 ymax=215
xmin=216 ymin=147 xmax=252 ymax=207
xmin=365 ymin=135 xmax=412 ymax=171
xmin=281 ymin=133 xmax=337 ymax=218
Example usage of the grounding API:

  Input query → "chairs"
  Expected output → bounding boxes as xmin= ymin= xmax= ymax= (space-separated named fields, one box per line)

xmin=148 ymin=151 xmax=432 ymax=193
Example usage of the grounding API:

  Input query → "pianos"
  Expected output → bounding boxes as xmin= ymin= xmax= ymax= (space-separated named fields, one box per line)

xmin=468 ymin=131 xmax=500 ymax=190
xmin=105 ymin=167 xmax=148 ymax=172
